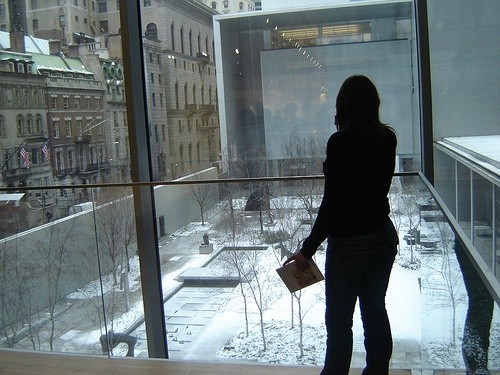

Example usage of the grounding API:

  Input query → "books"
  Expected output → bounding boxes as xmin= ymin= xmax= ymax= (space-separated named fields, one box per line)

xmin=276 ymin=258 xmax=324 ymax=294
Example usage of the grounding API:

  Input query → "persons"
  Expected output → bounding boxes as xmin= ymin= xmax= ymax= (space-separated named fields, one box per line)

xmin=282 ymin=75 xmax=399 ymax=375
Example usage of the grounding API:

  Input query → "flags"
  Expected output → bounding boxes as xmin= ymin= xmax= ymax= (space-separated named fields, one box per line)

xmin=41 ymin=145 xmax=49 ymax=162
xmin=21 ymin=147 xmax=30 ymax=168
